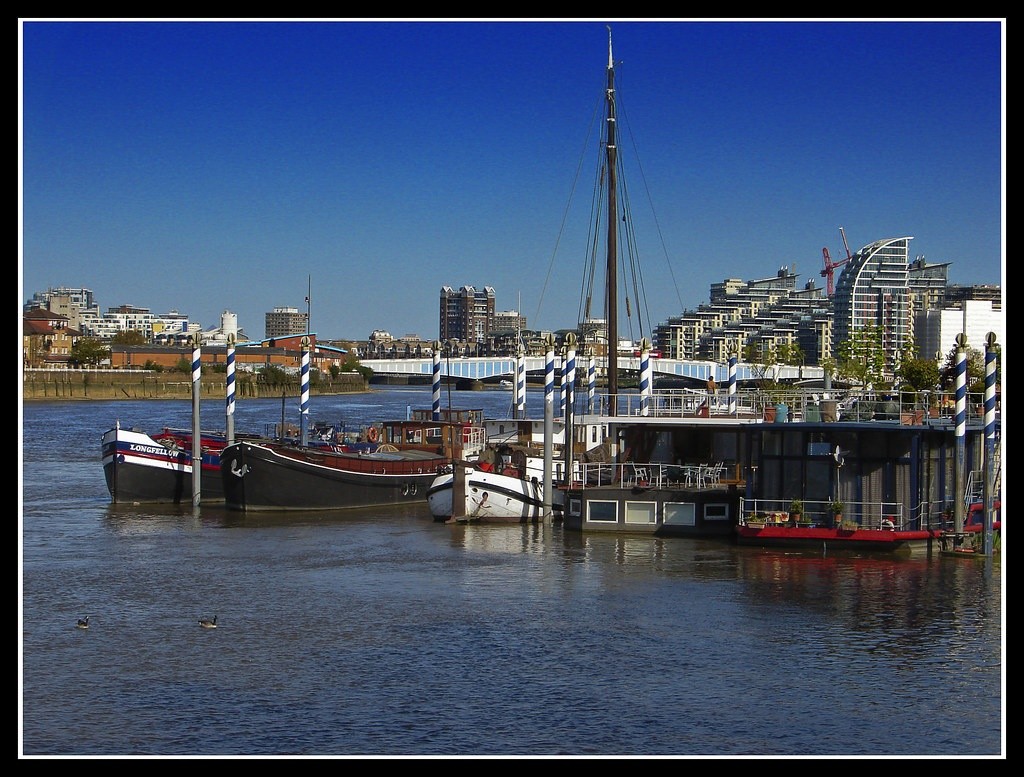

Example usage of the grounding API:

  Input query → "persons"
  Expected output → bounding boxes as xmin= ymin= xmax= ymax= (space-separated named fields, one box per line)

xmin=788 ymin=403 xmax=793 ymax=422
xmin=706 ymin=376 xmax=715 ymax=405
xmin=881 ymin=393 xmax=892 ymax=402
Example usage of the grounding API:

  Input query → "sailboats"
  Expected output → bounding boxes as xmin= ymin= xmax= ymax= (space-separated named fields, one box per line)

xmin=427 ymin=26 xmax=708 ymax=520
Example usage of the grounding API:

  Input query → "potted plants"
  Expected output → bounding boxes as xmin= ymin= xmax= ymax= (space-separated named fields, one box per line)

xmin=841 ymin=521 xmax=858 ymax=531
xmin=831 ymin=501 xmax=845 ymax=522
xmin=745 ymin=514 xmax=767 ymax=529
xmin=899 ymin=386 xmax=925 ymax=425
xmin=790 ymin=498 xmax=803 ymax=521
xmin=942 ymin=400 xmax=957 ymax=420
xmin=750 ymin=349 xmax=778 ymax=422
xmin=928 ymin=387 xmax=940 ymax=419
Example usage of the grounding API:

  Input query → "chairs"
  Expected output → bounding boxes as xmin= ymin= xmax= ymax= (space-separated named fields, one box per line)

xmin=625 ymin=461 xmax=723 ymax=490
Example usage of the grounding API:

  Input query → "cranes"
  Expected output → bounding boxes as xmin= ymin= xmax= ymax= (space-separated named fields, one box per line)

xmin=820 ymin=226 xmax=854 ymax=296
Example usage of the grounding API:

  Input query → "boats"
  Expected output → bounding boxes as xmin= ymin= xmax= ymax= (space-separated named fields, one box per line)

xmin=99 ymin=421 xmax=382 ymax=505
xmin=939 ymin=533 xmax=986 ymax=558
xmin=495 ymin=380 xmax=513 ymax=391
xmin=220 ymin=408 xmax=484 ymax=511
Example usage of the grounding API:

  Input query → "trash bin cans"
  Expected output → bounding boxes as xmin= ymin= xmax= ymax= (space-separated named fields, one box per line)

xmin=819 ymin=401 xmax=837 ymax=423
xmin=774 ymin=404 xmax=788 ymax=423
xmin=805 ymin=405 xmax=821 ymax=422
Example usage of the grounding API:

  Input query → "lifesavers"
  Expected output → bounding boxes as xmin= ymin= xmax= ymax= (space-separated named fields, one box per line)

xmin=367 ymin=426 xmax=379 ymax=442
xmin=955 ymin=547 xmax=974 ymax=553
